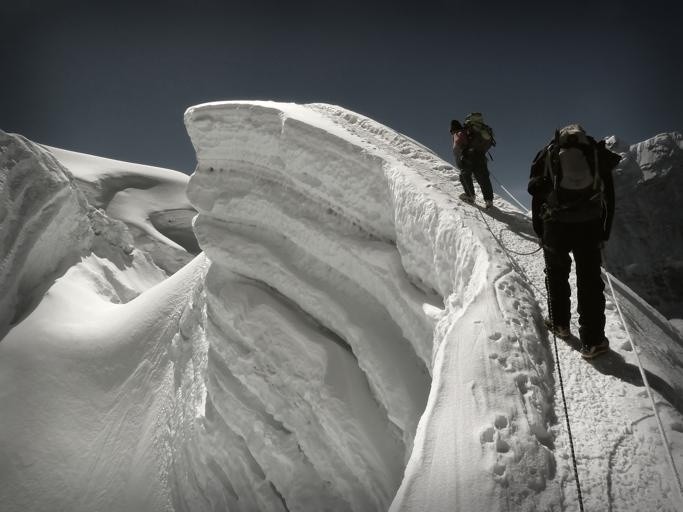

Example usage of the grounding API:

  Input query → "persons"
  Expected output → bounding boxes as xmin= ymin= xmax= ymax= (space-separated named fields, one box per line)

xmin=451 ymin=112 xmax=496 ymax=209
xmin=529 ymin=123 xmax=622 ymax=358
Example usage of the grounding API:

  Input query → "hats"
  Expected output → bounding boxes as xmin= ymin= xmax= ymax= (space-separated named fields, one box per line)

xmin=558 ymin=125 xmax=589 ymax=146
xmin=450 ymin=120 xmax=462 ymax=132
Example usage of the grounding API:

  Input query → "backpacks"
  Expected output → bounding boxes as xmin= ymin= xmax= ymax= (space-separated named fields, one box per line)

xmin=537 ymin=143 xmax=609 ymax=247
xmin=463 ymin=112 xmax=496 ymax=161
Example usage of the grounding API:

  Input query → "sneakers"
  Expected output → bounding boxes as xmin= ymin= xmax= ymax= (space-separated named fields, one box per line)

xmin=581 ymin=337 xmax=609 ymax=358
xmin=459 ymin=193 xmax=476 ymax=202
xmin=485 ymin=199 xmax=493 ymax=206
xmin=545 ymin=314 xmax=571 ymax=338
